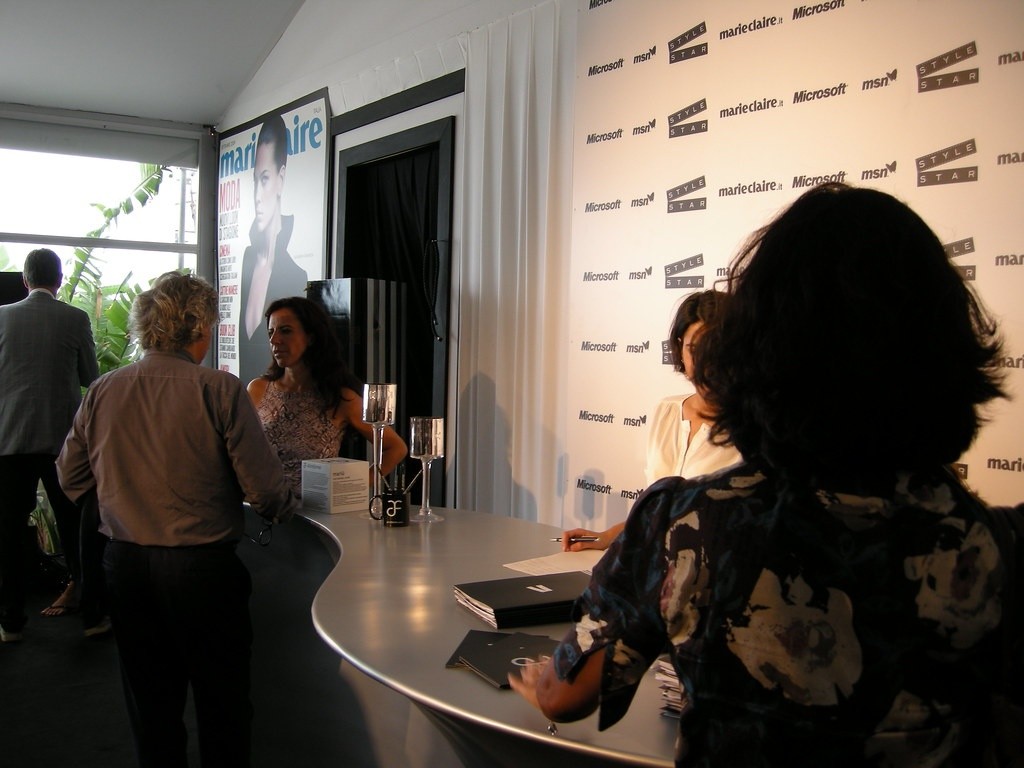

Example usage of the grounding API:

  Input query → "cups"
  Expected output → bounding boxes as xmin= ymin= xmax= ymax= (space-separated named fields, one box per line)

xmin=368 ymin=490 xmax=410 ymax=527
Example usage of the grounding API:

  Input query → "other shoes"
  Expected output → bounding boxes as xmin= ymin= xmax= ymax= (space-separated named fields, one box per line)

xmin=1 ymin=624 xmax=24 ymax=641
xmin=83 ymin=617 xmax=112 ymax=636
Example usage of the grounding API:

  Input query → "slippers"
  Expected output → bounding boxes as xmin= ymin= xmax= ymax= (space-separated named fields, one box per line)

xmin=41 ymin=604 xmax=76 ymax=616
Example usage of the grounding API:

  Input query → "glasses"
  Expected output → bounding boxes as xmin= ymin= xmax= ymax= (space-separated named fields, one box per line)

xmin=244 ymin=517 xmax=274 ymax=546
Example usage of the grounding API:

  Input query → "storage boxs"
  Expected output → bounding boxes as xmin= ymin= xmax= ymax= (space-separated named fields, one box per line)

xmin=301 ymin=457 xmax=369 ymax=514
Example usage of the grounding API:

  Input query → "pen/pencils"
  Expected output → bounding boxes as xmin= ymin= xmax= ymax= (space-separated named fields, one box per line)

xmin=549 ymin=536 xmax=600 ymax=542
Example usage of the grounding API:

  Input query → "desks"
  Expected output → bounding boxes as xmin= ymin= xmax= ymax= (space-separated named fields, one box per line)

xmin=290 ymin=500 xmax=681 ymax=768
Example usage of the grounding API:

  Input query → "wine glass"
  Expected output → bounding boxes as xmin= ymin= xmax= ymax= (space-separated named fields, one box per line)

xmin=408 ymin=415 xmax=445 ymax=523
xmin=357 ymin=382 xmax=397 ymax=520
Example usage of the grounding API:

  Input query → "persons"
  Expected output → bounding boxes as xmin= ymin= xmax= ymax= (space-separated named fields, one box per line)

xmin=246 ymin=297 xmax=406 ymax=489
xmin=560 ymin=290 xmax=746 ymax=551
xmin=237 ymin=115 xmax=307 ymax=391
xmin=55 ymin=272 xmax=301 ymax=768
xmin=0 ymin=248 xmax=112 ymax=640
xmin=506 ymin=179 xmax=1024 ymax=768
